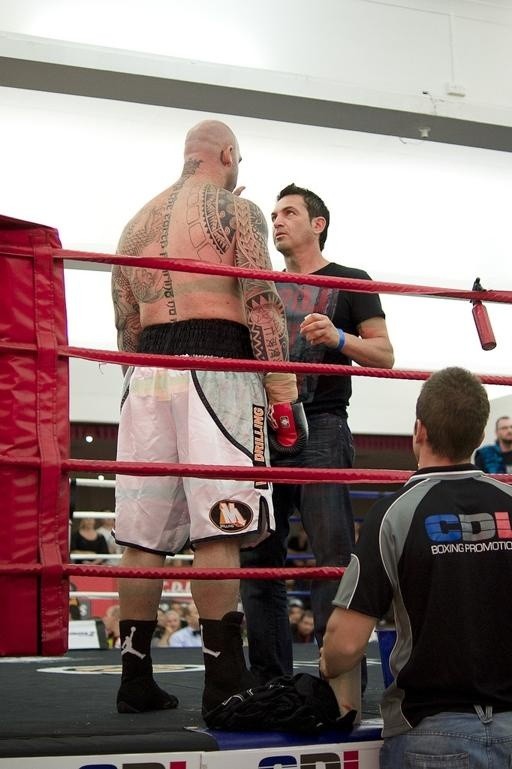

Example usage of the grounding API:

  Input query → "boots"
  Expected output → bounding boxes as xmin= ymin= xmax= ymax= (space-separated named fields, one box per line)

xmin=117 ymin=619 xmax=179 ymax=714
xmin=199 ymin=611 xmax=253 ymax=715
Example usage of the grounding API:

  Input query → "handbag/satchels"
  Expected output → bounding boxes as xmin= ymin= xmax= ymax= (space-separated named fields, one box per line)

xmin=203 ymin=672 xmax=356 ymax=740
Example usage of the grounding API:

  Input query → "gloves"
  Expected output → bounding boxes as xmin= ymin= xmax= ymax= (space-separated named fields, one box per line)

xmin=267 ymin=401 xmax=310 ymax=459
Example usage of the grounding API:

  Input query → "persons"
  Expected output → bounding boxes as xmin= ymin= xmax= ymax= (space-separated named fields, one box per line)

xmin=473 ymin=415 xmax=511 ymax=476
xmin=232 ymin=182 xmax=397 ymax=697
xmin=318 ymin=365 xmax=511 ymax=769
xmin=109 ymin=118 xmax=311 ymax=715
xmin=70 ymin=509 xmax=316 ymax=649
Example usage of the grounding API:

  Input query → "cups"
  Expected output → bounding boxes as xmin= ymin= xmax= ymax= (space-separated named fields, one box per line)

xmin=375 ymin=628 xmax=397 ymax=690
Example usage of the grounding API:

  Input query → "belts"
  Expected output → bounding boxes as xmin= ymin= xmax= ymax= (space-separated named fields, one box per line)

xmin=440 ymin=704 xmax=512 ymax=714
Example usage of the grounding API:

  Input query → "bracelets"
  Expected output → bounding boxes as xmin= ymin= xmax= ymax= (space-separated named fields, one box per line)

xmin=324 ymin=327 xmax=345 ymax=354
